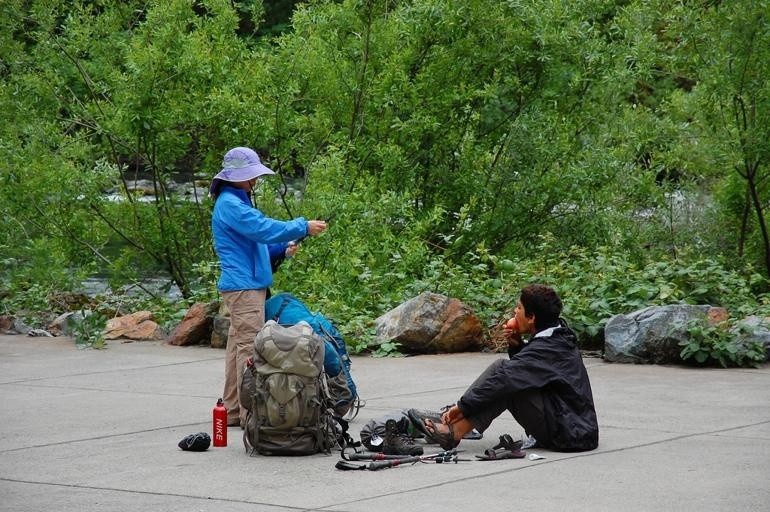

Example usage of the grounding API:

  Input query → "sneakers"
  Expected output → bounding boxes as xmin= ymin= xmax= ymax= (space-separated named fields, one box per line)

xmin=384 ymin=430 xmax=423 ymax=455
xmin=409 ymin=408 xmax=443 ymax=444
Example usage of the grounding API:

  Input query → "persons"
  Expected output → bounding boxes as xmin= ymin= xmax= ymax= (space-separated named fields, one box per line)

xmin=418 ymin=285 xmax=600 ymax=450
xmin=205 ymin=146 xmax=328 ymax=429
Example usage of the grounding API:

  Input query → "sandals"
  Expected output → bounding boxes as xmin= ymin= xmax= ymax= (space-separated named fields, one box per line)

xmin=475 ymin=433 xmax=526 ymax=460
xmin=420 ymin=418 xmax=461 ymax=450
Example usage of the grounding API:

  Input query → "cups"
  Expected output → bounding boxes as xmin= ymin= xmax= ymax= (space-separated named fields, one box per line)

xmin=212 ymin=399 xmax=226 ymax=446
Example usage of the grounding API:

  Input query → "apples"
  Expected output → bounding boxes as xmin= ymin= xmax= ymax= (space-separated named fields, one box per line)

xmin=506 ymin=318 xmax=518 ymax=335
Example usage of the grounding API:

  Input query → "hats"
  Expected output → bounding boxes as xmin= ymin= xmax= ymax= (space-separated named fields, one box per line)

xmin=210 ymin=147 xmax=276 ymax=194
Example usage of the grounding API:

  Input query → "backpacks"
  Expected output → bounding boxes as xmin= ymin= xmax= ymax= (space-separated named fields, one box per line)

xmin=238 ymin=320 xmax=341 ymax=456
xmin=264 ymin=293 xmax=357 ymax=410
